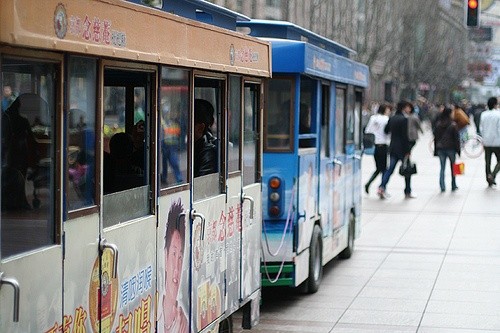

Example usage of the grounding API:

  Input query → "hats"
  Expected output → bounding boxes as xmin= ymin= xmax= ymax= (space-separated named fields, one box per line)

xmin=193 ymin=98 xmax=215 ymax=126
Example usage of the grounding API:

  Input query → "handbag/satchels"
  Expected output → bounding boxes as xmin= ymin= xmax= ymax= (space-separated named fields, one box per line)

xmin=452 ymin=159 xmax=465 ymax=175
xmin=399 ymin=156 xmax=417 ymax=177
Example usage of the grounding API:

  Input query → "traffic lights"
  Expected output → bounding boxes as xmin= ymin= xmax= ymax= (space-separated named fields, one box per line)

xmin=466 ymin=0 xmax=478 ymax=27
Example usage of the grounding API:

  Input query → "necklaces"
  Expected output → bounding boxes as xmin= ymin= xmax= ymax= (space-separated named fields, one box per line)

xmin=164 ymin=319 xmax=174 ymax=330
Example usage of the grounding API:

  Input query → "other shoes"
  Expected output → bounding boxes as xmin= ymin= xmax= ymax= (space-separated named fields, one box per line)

xmin=488 ymin=174 xmax=496 ymax=186
xmin=377 ymin=186 xmax=388 ymax=200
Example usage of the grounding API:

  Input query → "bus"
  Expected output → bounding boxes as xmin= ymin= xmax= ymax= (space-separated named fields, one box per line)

xmin=0 ymin=0 xmax=369 ymax=333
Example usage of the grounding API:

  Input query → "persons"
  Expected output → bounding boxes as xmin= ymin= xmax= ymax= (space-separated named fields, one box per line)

xmin=157 ymin=197 xmax=189 ymax=333
xmin=305 ymin=164 xmax=317 ymax=217
xmin=0 ymin=87 xmax=500 ymax=208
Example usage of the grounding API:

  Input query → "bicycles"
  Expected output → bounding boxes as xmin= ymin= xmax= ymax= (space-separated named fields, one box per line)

xmin=459 ymin=124 xmax=485 ymax=158
